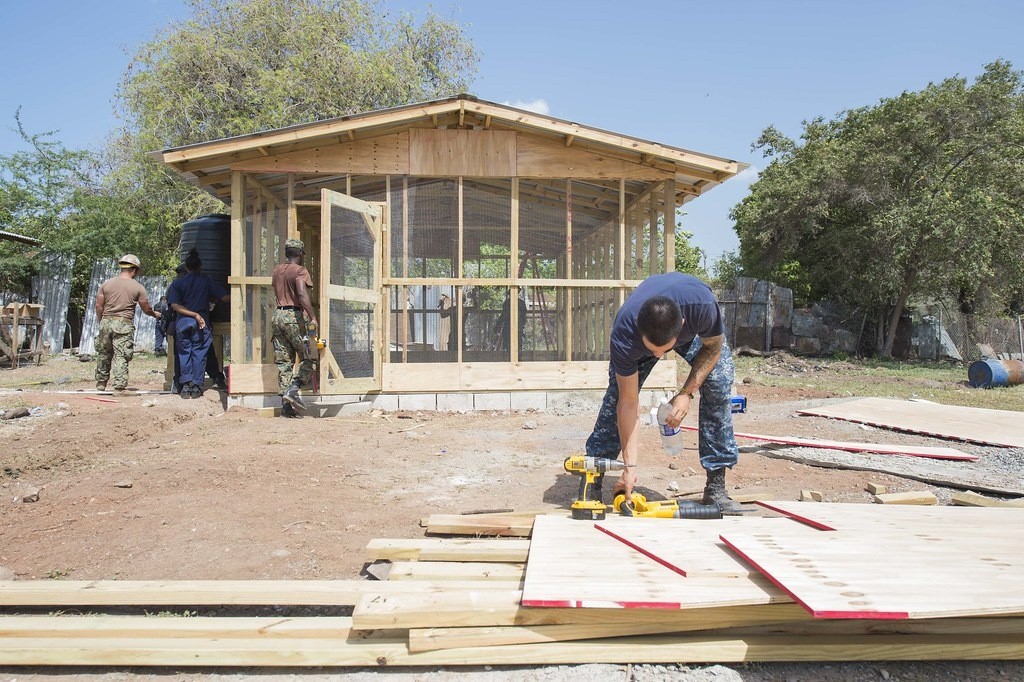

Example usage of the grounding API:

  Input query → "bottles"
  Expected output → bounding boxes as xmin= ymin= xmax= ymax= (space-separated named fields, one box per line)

xmin=657 ymin=398 xmax=684 ymax=456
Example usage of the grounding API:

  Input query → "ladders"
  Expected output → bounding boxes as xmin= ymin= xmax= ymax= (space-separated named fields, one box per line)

xmin=490 ymin=248 xmax=558 ymax=352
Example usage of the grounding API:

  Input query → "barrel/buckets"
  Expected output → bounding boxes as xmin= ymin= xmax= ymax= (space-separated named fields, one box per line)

xmin=968 ymin=359 xmax=1024 ymax=387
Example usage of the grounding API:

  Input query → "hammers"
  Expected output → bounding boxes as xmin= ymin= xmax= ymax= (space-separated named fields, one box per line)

xmin=438 ymin=294 xmax=449 ymax=308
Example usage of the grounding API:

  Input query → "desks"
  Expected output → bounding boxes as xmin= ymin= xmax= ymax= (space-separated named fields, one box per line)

xmin=0 ymin=315 xmax=46 ymax=370
xmin=162 ymin=321 xmax=231 ymax=392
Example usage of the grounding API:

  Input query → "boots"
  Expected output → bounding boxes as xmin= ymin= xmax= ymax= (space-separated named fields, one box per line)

xmin=280 ymin=399 xmax=303 ymax=418
xmin=703 ymin=470 xmax=742 ymax=515
xmin=217 ymin=381 xmax=228 ymax=390
xmin=578 ymin=471 xmax=605 ymax=502
xmin=172 ymin=381 xmax=181 ymax=393
xmin=283 ymin=378 xmax=308 ymax=411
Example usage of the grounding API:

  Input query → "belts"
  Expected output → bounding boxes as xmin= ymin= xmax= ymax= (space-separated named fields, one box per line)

xmin=277 ymin=305 xmax=303 ymax=309
xmin=104 ymin=316 xmax=132 ymax=322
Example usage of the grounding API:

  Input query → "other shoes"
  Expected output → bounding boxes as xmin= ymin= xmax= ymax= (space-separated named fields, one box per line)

xmin=113 ymin=388 xmax=125 ymax=394
xmin=191 ymin=386 xmax=204 ymax=399
xmin=180 ymin=385 xmax=191 ymax=400
xmin=95 ymin=382 xmax=108 ymax=390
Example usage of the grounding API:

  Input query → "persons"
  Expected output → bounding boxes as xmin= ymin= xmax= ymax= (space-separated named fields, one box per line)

xmin=154 ymin=295 xmax=169 ymax=352
xmin=94 ymin=254 xmax=162 ymax=391
xmin=439 ymin=294 xmax=479 ymax=351
xmin=165 ymin=247 xmax=229 ymax=399
xmin=580 ymin=270 xmax=746 ymax=515
xmin=271 ymin=239 xmax=319 ymax=417
xmin=495 ymin=285 xmax=526 ymax=361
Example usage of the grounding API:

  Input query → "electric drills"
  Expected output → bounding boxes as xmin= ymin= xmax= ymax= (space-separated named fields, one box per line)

xmin=614 ymin=490 xmax=760 ymax=519
xmin=564 ymin=455 xmax=638 ymax=520
xmin=302 ymin=322 xmax=326 ymax=349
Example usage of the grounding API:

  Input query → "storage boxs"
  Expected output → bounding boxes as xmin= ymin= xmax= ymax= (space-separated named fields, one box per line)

xmin=0 ymin=305 xmax=7 ymax=315
xmin=731 ymin=396 xmax=748 ymax=414
xmin=6 ymin=300 xmax=45 ymax=318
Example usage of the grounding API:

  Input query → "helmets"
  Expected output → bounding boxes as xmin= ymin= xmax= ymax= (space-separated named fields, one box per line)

xmin=285 ymin=239 xmax=307 ymax=255
xmin=119 ymin=254 xmax=141 ymax=269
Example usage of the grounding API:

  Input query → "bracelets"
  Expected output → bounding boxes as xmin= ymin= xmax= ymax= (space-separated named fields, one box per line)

xmin=679 ymin=387 xmax=694 ymax=399
